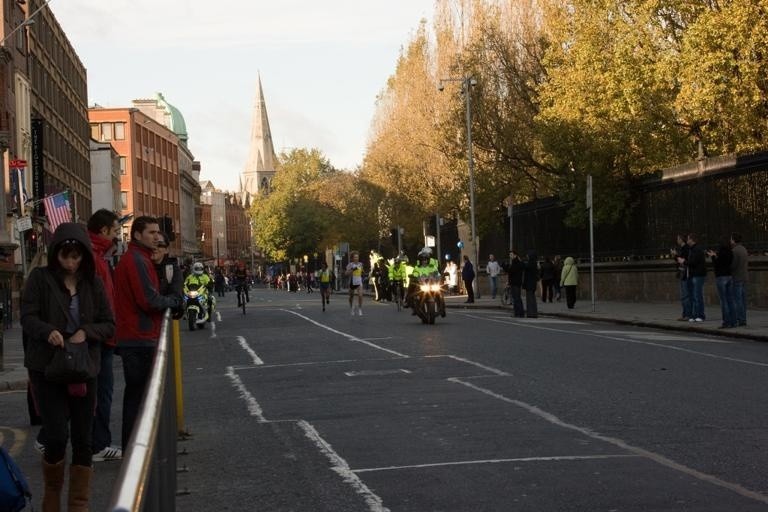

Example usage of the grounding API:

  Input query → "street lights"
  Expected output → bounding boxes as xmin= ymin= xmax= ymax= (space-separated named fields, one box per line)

xmin=0 ymin=19 xmax=36 ymax=45
xmin=438 ymin=70 xmax=486 ymax=298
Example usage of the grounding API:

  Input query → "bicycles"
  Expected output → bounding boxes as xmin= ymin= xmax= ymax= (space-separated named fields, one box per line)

xmin=231 ymin=275 xmax=250 ymax=314
xmin=500 ymin=280 xmax=513 ymax=307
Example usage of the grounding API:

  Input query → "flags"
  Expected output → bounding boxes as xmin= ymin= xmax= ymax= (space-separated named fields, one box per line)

xmin=42 ymin=191 xmax=73 ymax=235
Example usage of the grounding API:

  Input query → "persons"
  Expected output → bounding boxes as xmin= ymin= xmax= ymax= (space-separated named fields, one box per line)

xmin=486 ymin=254 xmax=501 ymax=299
xmin=368 ymin=247 xmax=458 ymax=317
xmin=677 ymin=234 xmax=707 ymax=322
xmin=272 ymin=269 xmax=320 ymax=294
xmin=318 ymin=263 xmax=331 ymax=312
xmin=345 ymin=251 xmax=366 ymax=316
xmin=114 ymin=217 xmax=190 ymax=456
xmin=707 ymin=233 xmax=737 ymax=329
xmin=462 ymin=255 xmax=474 ymax=303
xmin=670 ymin=233 xmax=694 ymax=320
xmin=502 ymin=250 xmax=578 ymax=318
xmin=183 ymin=262 xmax=214 ymax=323
xmin=33 ymin=208 xmax=121 ymax=463
xmin=207 ymin=262 xmax=249 ymax=308
xmin=729 ymin=233 xmax=749 ymax=326
xmin=22 ymin=222 xmax=115 ymax=511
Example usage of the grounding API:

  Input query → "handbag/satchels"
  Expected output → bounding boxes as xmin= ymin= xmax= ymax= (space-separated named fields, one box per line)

xmin=27 ymin=331 xmax=101 ymax=377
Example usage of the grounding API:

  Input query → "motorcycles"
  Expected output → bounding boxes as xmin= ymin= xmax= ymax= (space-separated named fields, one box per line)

xmin=183 ymin=283 xmax=216 ymax=331
xmin=406 ymin=272 xmax=446 ymax=325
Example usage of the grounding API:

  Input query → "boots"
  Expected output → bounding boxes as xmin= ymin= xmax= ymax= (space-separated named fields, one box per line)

xmin=68 ymin=463 xmax=95 ymax=512
xmin=41 ymin=459 xmax=65 ymax=512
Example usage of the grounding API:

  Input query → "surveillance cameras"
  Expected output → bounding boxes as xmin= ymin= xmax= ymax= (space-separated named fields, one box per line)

xmin=471 ymin=80 xmax=477 ymax=86
xmin=438 ymin=84 xmax=444 ymax=91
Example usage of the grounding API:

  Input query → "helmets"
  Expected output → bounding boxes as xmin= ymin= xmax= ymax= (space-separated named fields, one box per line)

xmin=192 ymin=262 xmax=204 ymax=275
xmin=418 ymin=251 xmax=430 ymax=264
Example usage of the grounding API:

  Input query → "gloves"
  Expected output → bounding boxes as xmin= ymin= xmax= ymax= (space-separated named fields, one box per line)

xmin=171 ymin=299 xmax=183 ymax=319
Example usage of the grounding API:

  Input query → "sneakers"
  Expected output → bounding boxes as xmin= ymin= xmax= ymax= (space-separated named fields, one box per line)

xmin=695 ymin=318 xmax=703 ymax=322
xmin=92 ymin=447 xmax=122 ymax=461
xmin=688 ymin=318 xmax=695 ymax=322
xmin=33 ymin=441 xmax=45 ymax=455
